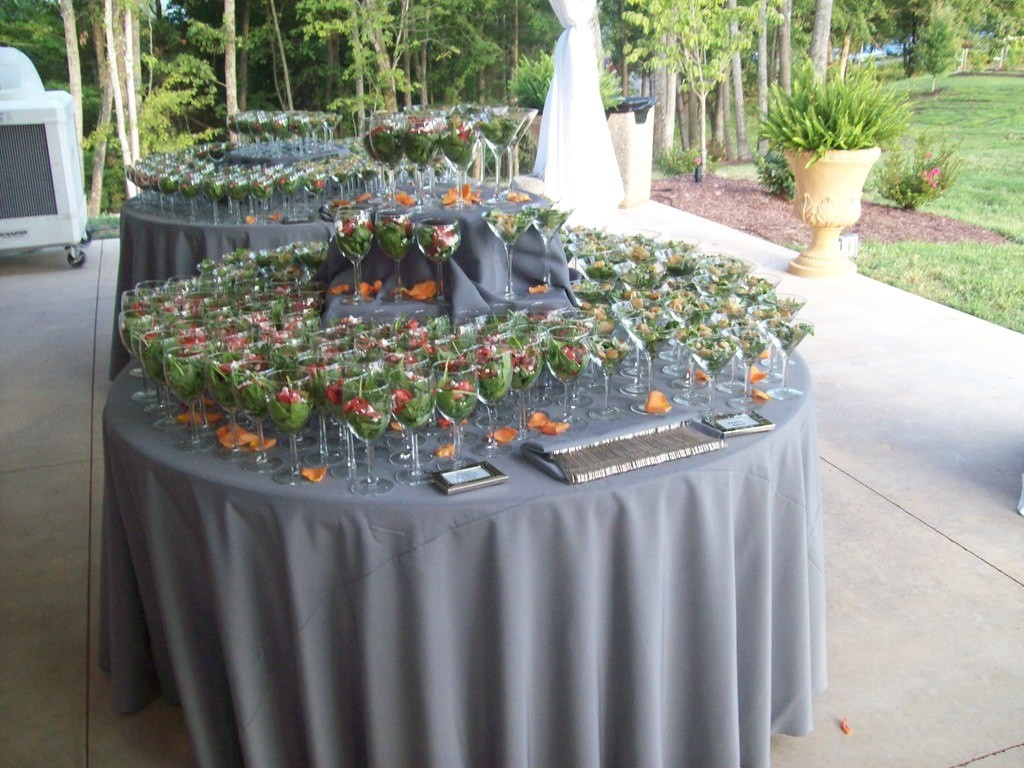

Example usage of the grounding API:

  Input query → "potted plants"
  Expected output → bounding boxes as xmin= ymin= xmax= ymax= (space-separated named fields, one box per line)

xmin=754 ymin=43 xmax=918 ymax=276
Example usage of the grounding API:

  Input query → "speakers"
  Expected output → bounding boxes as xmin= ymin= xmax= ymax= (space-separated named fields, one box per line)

xmin=0 ymin=46 xmax=88 ymax=251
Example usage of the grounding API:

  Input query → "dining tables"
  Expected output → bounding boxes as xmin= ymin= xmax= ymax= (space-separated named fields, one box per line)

xmin=101 ymin=346 xmax=824 ymax=765
xmin=107 ymin=183 xmax=553 ymax=383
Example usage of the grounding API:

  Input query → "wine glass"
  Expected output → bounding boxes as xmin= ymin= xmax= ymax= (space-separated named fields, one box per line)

xmin=119 ymin=104 xmax=813 ymax=496
